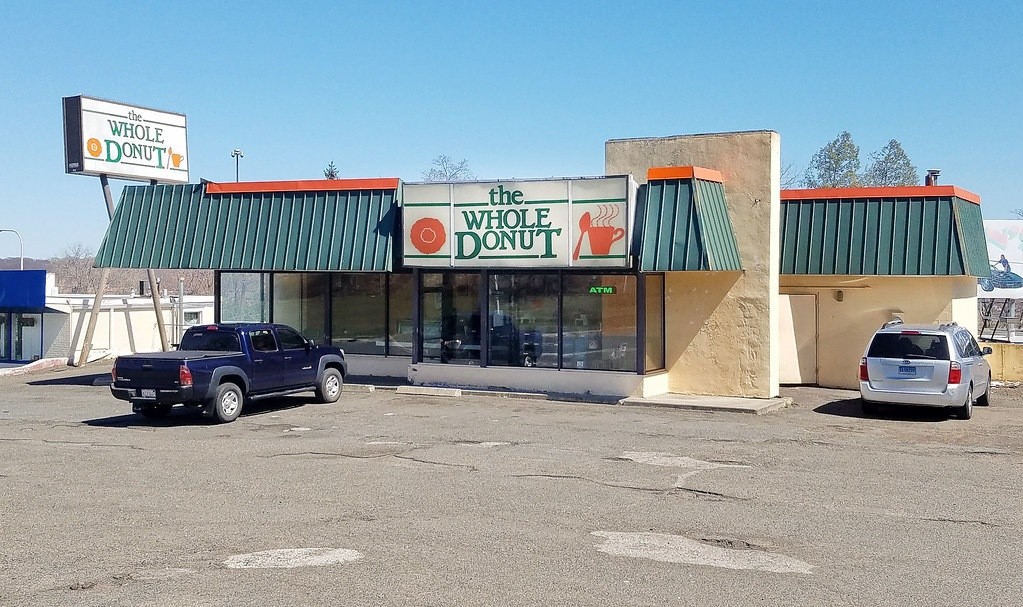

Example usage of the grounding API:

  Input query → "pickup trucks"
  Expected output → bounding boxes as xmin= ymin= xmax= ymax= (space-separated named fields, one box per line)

xmin=112 ymin=322 xmax=349 ymax=422
xmin=426 ymin=311 xmax=546 ymax=367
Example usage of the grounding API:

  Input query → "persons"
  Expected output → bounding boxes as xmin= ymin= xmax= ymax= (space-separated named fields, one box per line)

xmin=994 ymin=253 xmax=1011 ymax=273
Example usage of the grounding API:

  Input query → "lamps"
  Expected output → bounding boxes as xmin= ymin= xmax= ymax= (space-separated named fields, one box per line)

xmin=837 ymin=290 xmax=843 ymax=301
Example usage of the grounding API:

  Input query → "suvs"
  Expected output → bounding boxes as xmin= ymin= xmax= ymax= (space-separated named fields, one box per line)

xmin=856 ymin=320 xmax=992 ymax=418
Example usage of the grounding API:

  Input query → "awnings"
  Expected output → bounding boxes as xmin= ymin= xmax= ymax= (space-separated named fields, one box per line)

xmin=91 ymin=181 xmax=410 ymax=273
xmin=639 ymin=165 xmax=744 ymax=271
xmin=779 ymin=198 xmax=991 ymax=277
